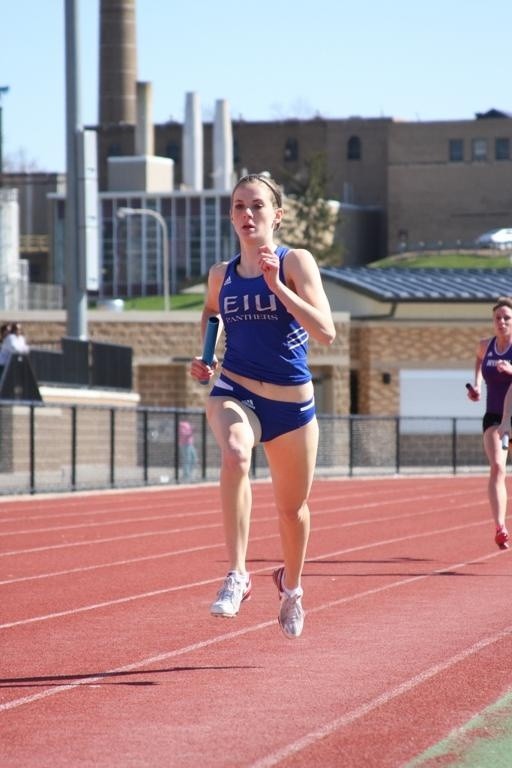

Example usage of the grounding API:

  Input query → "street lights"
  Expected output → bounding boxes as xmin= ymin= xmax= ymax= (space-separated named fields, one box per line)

xmin=118 ymin=208 xmax=172 ymax=312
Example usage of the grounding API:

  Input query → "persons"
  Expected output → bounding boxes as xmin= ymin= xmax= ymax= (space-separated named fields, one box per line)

xmin=0 ymin=323 xmax=12 ymax=343
xmin=179 ymin=414 xmax=198 ymax=479
xmin=189 ymin=172 xmax=338 ymax=640
xmin=0 ymin=322 xmax=29 ymax=377
xmin=465 ymin=296 xmax=512 ymax=551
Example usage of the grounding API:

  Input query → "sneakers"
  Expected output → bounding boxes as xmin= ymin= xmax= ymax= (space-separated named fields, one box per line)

xmin=274 ymin=567 xmax=306 ymax=640
xmin=496 ymin=524 xmax=508 ymax=551
xmin=212 ymin=569 xmax=252 ymax=618
